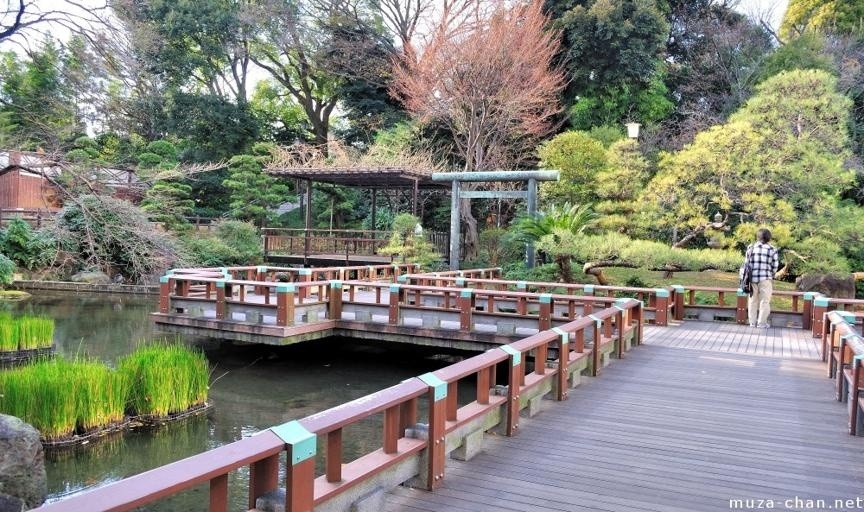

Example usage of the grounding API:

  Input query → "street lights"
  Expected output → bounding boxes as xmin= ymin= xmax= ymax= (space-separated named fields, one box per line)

xmin=624 ymin=121 xmax=642 ymax=150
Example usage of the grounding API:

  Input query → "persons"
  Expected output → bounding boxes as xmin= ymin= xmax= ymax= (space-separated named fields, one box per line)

xmin=741 ymin=228 xmax=779 ymax=331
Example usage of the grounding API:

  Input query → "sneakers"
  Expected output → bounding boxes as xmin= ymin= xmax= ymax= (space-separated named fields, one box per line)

xmin=749 ymin=321 xmax=757 ymax=328
xmin=758 ymin=322 xmax=771 ymax=328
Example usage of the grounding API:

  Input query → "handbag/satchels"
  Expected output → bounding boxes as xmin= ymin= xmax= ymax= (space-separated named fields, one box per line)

xmin=741 ymin=243 xmax=754 ymax=293
